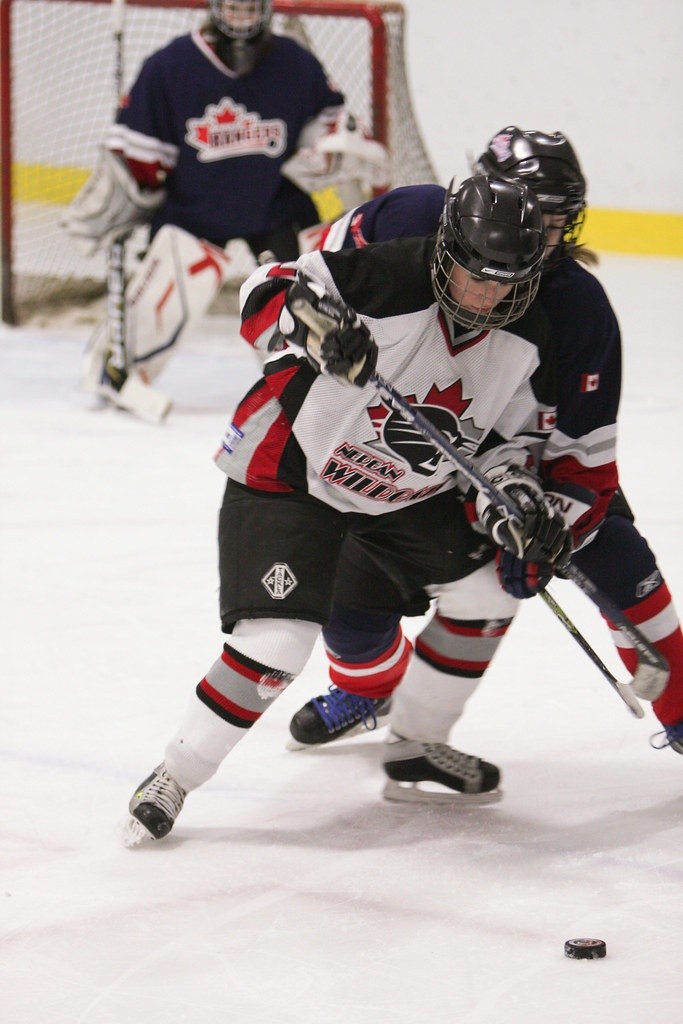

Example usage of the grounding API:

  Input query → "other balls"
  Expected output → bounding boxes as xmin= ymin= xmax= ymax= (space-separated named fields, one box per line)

xmin=563 ymin=938 xmax=609 ymax=960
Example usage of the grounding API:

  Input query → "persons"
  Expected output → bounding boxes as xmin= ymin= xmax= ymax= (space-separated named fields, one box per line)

xmin=121 ymin=124 xmax=683 ymax=849
xmin=59 ymin=0 xmax=394 ymax=425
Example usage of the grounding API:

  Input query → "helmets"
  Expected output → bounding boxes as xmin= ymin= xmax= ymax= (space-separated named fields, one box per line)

xmin=429 ymin=172 xmax=547 ymax=330
xmin=208 ymin=0 xmax=273 ymax=36
xmin=473 ymin=127 xmax=584 ymax=266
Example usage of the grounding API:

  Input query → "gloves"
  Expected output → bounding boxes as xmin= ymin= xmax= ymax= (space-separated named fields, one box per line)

xmin=494 ymin=480 xmax=608 ymax=598
xmin=279 ymin=284 xmax=377 ymax=387
xmin=476 ymin=481 xmax=573 ymax=569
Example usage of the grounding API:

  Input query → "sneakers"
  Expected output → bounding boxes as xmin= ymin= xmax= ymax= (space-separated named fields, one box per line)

xmin=383 ymin=729 xmax=502 ymax=804
xmin=125 ymin=762 xmax=187 ymax=848
xmin=286 ymin=682 xmax=390 ymax=750
xmin=649 ymin=714 xmax=683 ymax=758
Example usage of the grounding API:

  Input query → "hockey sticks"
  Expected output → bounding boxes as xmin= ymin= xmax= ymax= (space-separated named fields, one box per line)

xmin=539 ymin=589 xmax=644 ymax=719
xmin=369 ymin=373 xmax=671 ymax=700
xmin=87 ymin=1 xmax=174 ymax=421
